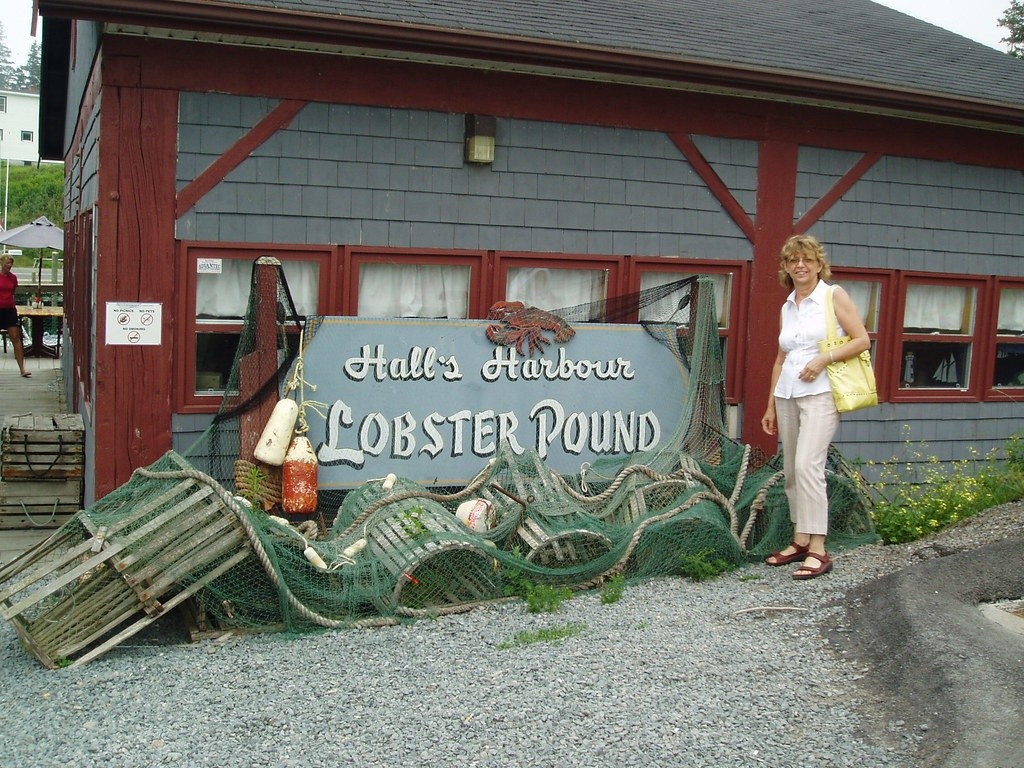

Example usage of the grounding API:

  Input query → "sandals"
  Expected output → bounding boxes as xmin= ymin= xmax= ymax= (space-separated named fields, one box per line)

xmin=766 ymin=542 xmax=810 ymax=567
xmin=792 ymin=550 xmax=833 ymax=580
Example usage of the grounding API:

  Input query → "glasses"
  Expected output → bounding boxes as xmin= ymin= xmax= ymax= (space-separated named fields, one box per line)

xmin=789 ymin=258 xmax=818 ymax=266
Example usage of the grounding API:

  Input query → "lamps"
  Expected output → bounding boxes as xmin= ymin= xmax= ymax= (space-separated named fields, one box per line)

xmin=462 ymin=111 xmax=497 ymax=165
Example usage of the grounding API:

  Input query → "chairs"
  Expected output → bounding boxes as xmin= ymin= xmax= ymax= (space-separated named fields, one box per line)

xmin=0 ymin=325 xmax=19 ymax=353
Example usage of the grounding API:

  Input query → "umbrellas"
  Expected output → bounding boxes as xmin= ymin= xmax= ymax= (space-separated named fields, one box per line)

xmin=0 ymin=215 xmax=64 ymax=299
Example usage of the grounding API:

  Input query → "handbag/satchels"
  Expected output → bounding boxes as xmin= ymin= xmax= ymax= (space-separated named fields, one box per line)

xmin=817 ymin=284 xmax=879 ymax=413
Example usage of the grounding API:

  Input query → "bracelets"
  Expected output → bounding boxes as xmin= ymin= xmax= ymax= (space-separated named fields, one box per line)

xmin=829 ymin=351 xmax=834 ymax=363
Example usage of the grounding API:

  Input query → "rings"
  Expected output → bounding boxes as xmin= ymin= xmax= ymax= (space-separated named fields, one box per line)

xmin=811 ymin=376 xmax=815 ymax=380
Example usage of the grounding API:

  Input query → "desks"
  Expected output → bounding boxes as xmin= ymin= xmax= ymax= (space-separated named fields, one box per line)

xmin=15 ymin=305 xmax=64 ymax=359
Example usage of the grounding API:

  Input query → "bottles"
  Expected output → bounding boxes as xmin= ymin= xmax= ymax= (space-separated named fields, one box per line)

xmin=282 ymin=437 xmax=318 ymax=514
xmin=253 ymin=398 xmax=299 ymax=464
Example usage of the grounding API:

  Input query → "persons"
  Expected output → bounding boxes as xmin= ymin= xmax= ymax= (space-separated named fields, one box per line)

xmin=0 ymin=254 xmax=32 ymax=377
xmin=761 ymin=233 xmax=872 ymax=580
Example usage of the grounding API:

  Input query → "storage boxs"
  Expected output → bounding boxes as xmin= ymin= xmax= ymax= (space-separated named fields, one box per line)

xmin=0 ymin=412 xmax=85 ymax=531
